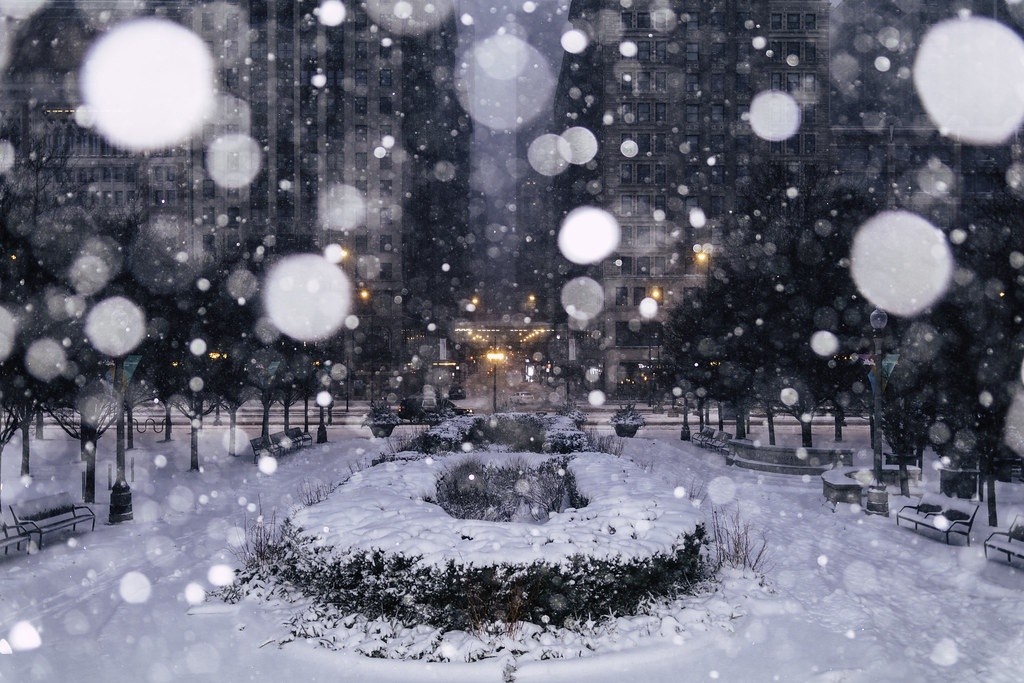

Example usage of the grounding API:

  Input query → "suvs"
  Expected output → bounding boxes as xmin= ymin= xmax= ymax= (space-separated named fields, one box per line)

xmin=509 ymin=392 xmax=535 ymax=405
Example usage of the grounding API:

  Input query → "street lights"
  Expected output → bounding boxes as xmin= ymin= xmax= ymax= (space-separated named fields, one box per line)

xmin=868 ymin=305 xmax=889 ymax=513
xmin=486 ymin=337 xmax=505 ymax=413
xmin=108 ymin=304 xmax=133 ymax=523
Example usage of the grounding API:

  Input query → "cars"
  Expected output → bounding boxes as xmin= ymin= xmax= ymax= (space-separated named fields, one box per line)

xmin=398 ymin=397 xmax=473 ymax=424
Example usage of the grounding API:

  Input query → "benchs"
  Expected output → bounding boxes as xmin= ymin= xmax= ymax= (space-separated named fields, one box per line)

xmin=0 ymin=509 xmax=31 ymax=556
xmin=250 ymin=435 xmax=281 ymax=465
xmin=269 ymin=431 xmax=298 ymax=457
xmin=705 ymin=431 xmax=732 ymax=454
xmin=691 ymin=426 xmax=715 ymax=448
xmin=287 ymin=427 xmax=312 ymax=451
xmin=721 ymin=436 xmax=753 ymax=456
xmin=984 ymin=514 xmax=1024 ymax=563
xmin=8 ymin=492 xmax=96 ymax=550
xmin=897 ymin=492 xmax=980 ymax=546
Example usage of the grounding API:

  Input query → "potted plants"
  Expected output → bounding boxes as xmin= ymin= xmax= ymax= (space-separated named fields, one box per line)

xmin=361 ymin=400 xmax=401 ymax=438
xmin=609 ymin=396 xmax=647 ymax=437
